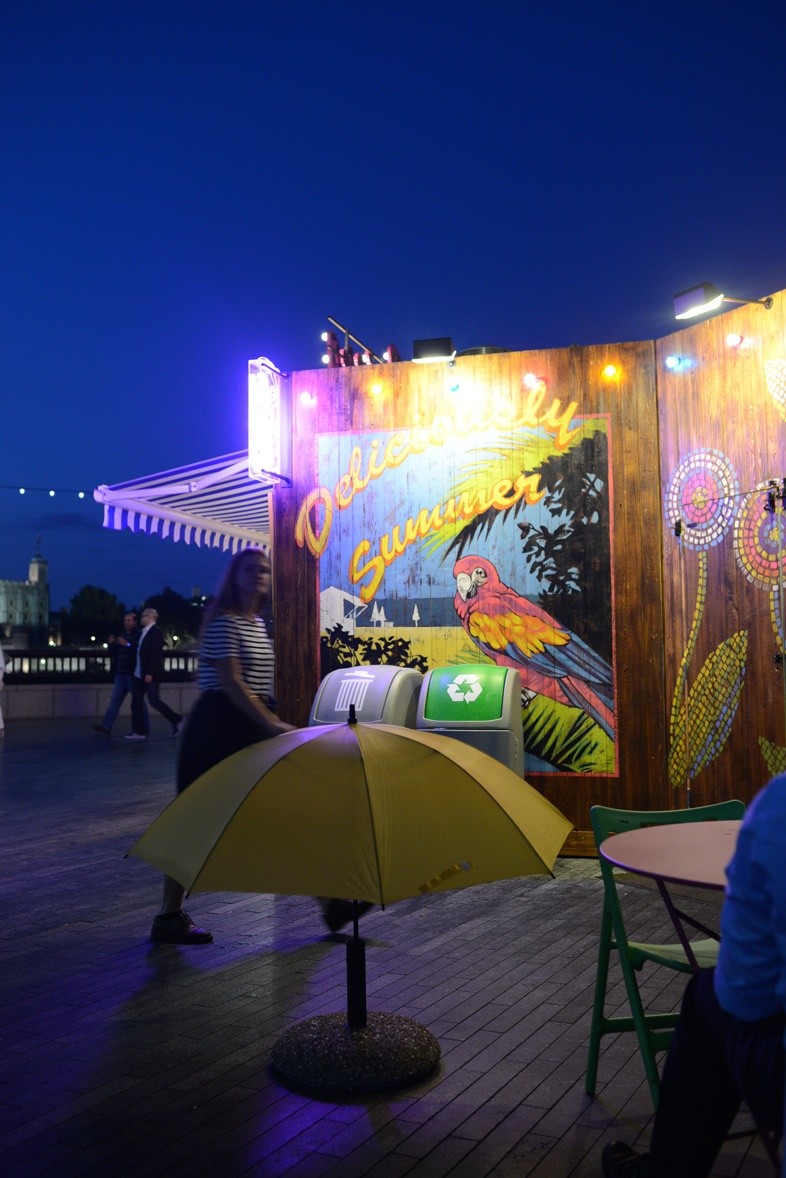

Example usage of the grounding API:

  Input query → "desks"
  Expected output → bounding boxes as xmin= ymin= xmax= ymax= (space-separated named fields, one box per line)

xmin=598 ymin=818 xmax=781 ymax=1004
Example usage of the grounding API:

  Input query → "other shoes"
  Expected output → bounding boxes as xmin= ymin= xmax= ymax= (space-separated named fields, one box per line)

xmin=172 ymin=715 xmax=188 ymax=738
xmin=149 ymin=909 xmax=216 ymax=946
xmin=323 ymin=896 xmax=376 ymax=935
xmin=601 ymin=1139 xmax=659 ymax=1178
xmin=123 ymin=732 xmax=148 ymax=742
xmin=95 ymin=727 xmax=111 ymax=736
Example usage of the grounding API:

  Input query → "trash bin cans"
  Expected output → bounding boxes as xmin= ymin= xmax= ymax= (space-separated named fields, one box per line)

xmin=416 ymin=664 xmax=524 ymax=781
xmin=309 ymin=665 xmax=424 ymax=731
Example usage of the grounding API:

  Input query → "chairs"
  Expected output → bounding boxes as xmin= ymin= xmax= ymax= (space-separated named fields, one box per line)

xmin=586 ymin=797 xmax=754 ymax=1104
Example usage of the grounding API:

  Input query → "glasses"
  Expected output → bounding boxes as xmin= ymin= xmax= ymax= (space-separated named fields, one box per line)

xmin=140 ymin=614 xmax=149 ymax=617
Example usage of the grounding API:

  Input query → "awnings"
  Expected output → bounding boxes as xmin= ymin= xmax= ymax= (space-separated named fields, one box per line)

xmin=90 ymin=447 xmax=272 ymax=559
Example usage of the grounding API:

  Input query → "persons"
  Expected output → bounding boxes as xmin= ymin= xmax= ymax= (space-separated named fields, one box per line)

xmin=89 ymin=612 xmax=151 ymax=737
xmin=599 ymin=767 xmax=786 ymax=1178
xmin=123 ymin=608 xmax=187 ymax=740
xmin=151 ymin=544 xmax=376 ymax=945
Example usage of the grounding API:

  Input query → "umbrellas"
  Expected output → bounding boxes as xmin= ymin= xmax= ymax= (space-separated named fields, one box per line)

xmin=121 ymin=703 xmax=577 ymax=1031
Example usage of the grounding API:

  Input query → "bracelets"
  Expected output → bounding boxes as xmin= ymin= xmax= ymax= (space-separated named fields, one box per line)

xmin=126 ymin=642 xmax=131 ymax=647
